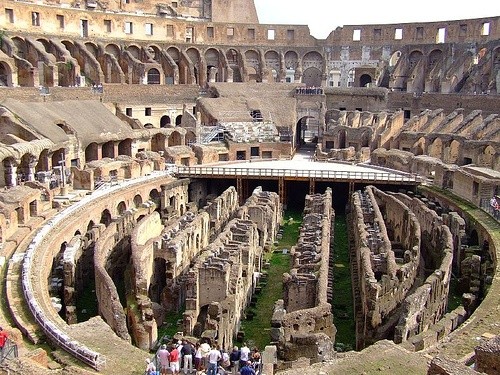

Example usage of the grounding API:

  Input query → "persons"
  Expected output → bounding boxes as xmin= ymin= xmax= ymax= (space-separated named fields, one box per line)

xmin=144 ymin=340 xmax=261 ymax=375
xmin=94 ymin=83 xmax=103 ymax=87
xmin=296 ymin=85 xmax=323 ymax=94
xmin=490 ymin=196 xmax=500 ymax=215
xmin=0 ymin=327 xmax=7 ymax=349
